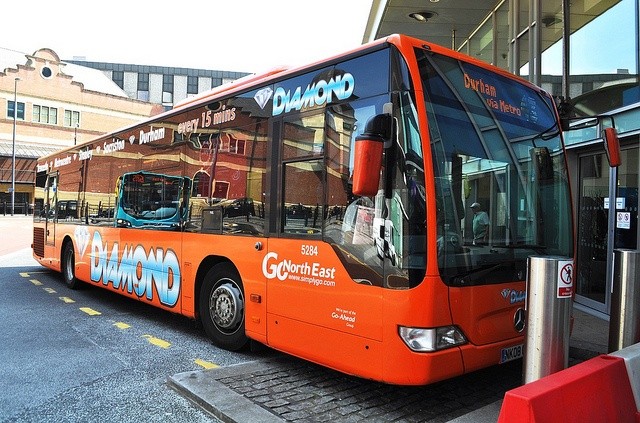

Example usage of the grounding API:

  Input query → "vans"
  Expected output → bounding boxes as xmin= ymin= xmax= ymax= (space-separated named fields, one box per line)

xmin=216 ymin=196 xmax=255 ymax=217
xmin=48 ymin=199 xmax=82 ymax=218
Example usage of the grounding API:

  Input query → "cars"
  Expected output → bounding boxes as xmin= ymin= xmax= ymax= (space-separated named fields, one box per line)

xmin=287 ymin=204 xmax=313 ymax=218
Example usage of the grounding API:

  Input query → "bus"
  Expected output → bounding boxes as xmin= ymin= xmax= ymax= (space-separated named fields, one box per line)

xmin=112 ymin=169 xmax=193 ymax=231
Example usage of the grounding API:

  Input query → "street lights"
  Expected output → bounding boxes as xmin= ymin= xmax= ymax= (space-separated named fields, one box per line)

xmin=30 ymin=32 xmax=622 ymax=386
xmin=10 ymin=77 xmax=23 ymax=215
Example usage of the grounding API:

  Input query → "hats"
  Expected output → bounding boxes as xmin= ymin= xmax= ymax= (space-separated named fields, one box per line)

xmin=468 ymin=203 xmax=480 ymax=208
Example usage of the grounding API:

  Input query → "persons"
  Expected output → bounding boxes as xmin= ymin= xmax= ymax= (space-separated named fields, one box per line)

xmin=470 ymin=202 xmax=490 ymax=245
